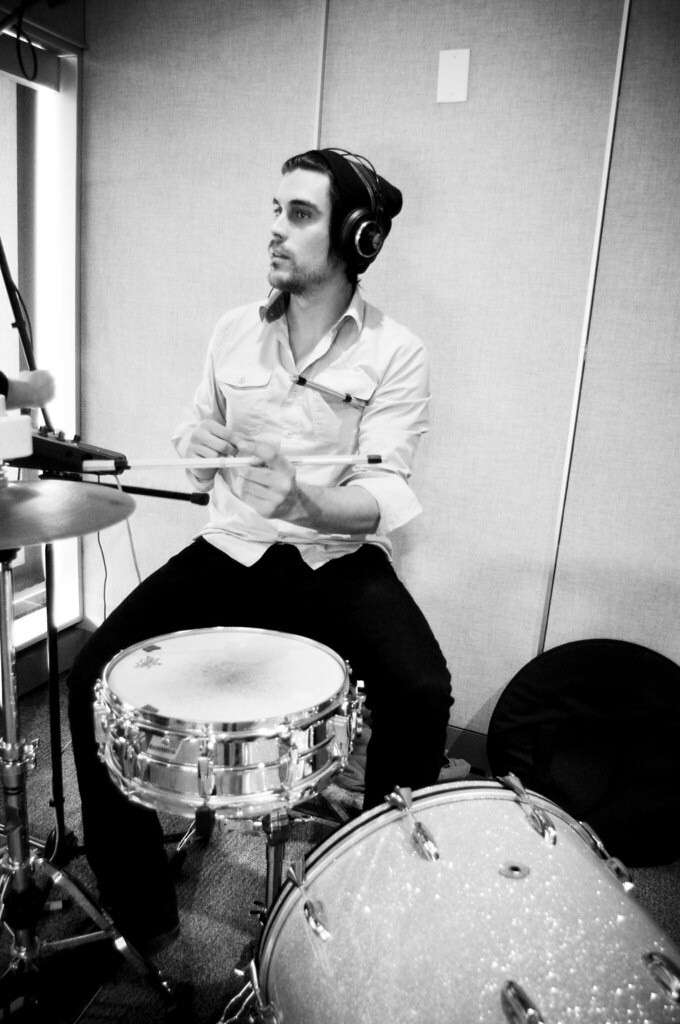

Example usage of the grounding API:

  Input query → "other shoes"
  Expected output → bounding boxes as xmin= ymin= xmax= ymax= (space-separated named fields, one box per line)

xmin=69 ymin=921 xmax=182 ymax=978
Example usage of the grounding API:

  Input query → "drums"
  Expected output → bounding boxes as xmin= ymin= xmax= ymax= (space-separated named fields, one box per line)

xmin=92 ymin=626 xmax=366 ymax=819
xmin=231 ymin=777 xmax=680 ymax=1024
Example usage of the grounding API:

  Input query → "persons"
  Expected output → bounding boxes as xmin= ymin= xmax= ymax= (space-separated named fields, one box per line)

xmin=67 ymin=148 xmax=452 ymax=951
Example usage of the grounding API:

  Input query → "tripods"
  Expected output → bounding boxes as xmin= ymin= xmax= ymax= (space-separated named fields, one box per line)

xmin=0 ymin=548 xmax=182 ymax=1024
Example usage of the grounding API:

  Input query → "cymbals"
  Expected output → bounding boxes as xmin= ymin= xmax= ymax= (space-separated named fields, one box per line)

xmin=1 ymin=478 xmax=137 ymax=552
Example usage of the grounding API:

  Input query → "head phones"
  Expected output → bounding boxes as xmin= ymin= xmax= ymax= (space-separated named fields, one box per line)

xmin=319 ymin=147 xmax=386 ymax=268
